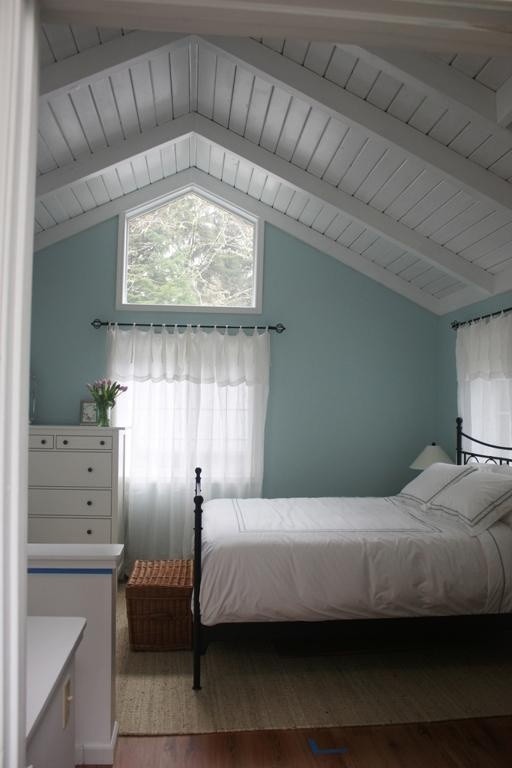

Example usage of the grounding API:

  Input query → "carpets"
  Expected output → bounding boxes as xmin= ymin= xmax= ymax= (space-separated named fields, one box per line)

xmin=114 ymin=582 xmax=512 ymax=737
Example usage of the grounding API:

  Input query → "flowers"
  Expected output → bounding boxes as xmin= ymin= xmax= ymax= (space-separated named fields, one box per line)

xmin=86 ymin=379 xmax=130 ymax=417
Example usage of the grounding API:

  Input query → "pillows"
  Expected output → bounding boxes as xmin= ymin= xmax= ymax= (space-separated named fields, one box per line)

xmin=392 ymin=462 xmax=512 ymax=537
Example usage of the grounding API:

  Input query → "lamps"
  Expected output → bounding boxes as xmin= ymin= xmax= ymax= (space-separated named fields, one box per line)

xmin=408 ymin=442 xmax=456 ymax=471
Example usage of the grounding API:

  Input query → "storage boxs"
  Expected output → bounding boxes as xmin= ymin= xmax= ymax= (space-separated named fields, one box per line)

xmin=123 ymin=557 xmax=194 ymax=651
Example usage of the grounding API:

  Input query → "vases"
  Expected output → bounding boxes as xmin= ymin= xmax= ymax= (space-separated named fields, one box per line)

xmin=95 ymin=404 xmax=112 ymax=428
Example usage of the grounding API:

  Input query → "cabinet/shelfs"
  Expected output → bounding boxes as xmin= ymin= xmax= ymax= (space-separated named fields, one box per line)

xmin=27 ymin=426 xmax=125 ymax=544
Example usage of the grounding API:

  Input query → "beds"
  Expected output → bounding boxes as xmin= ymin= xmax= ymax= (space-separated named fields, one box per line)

xmin=191 ymin=415 xmax=512 ymax=692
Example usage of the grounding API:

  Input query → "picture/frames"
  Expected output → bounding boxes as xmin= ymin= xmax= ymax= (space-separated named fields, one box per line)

xmin=79 ymin=400 xmax=97 ymax=425
